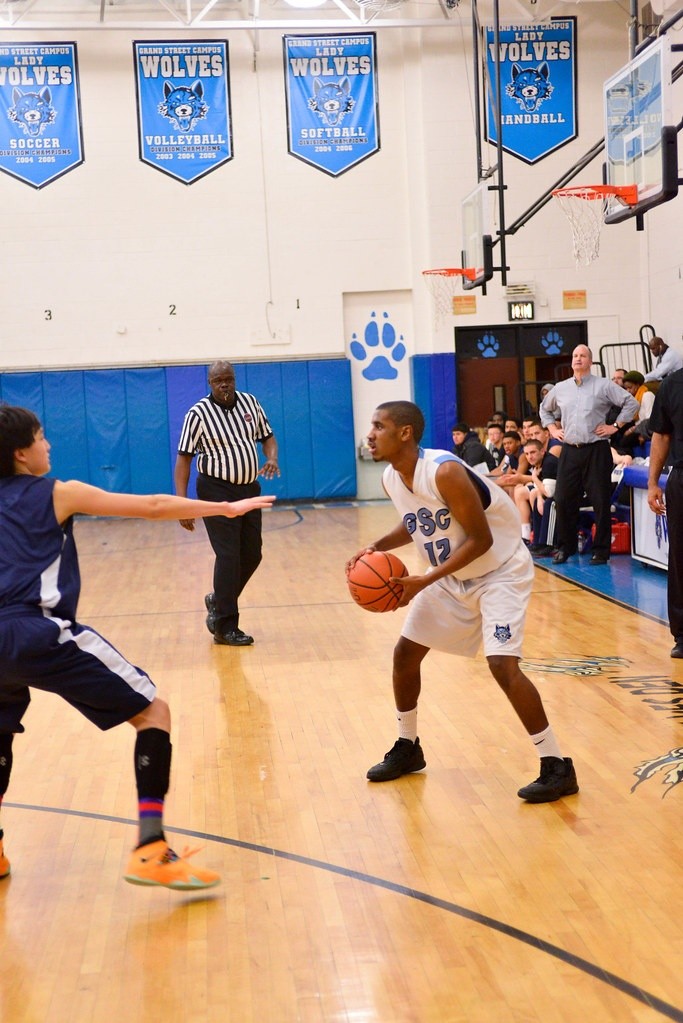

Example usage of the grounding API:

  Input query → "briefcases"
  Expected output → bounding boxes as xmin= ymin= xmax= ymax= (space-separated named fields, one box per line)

xmin=592 ymin=518 xmax=631 ymax=555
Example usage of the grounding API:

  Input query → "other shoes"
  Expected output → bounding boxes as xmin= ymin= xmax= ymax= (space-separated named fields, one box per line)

xmin=589 ymin=554 xmax=609 ymax=565
xmin=522 ymin=538 xmax=531 ymax=546
xmin=552 ymin=545 xmax=580 ymax=564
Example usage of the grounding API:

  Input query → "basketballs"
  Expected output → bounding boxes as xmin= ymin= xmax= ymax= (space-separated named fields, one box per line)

xmin=348 ymin=551 xmax=409 ymax=613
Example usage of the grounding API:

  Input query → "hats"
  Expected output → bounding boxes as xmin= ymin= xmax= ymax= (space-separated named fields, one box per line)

xmin=622 ymin=370 xmax=645 ymax=385
xmin=451 ymin=423 xmax=470 ymax=433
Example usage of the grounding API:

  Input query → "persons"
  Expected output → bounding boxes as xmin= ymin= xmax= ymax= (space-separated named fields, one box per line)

xmin=174 ymin=359 xmax=281 ymax=646
xmin=0 ymin=405 xmax=278 ymax=892
xmin=345 ymin=400 xmax=580 ymax=803
xmin=451 ymin=337 xmax=683 ymax=559
xmin=647 ymin=367 xmax=683 ymax=659
xmin=538 ymin=344 xmax=641 ymax=566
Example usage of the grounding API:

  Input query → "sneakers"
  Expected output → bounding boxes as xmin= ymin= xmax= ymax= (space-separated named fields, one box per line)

xmin=670 ymin=643 xmax=683 ymax=658
xmin=0 ymin=839 xmax=12 ymax=878
xmin=204 ymin=592 xmax=218 ymax=635
xmin=213 ymin=627 xmax=254 ymax=646
xmin=121 ymin=830 xmax=222 ymax=889
xmin=517 ymin=754 xmax=580 ymax=803
xmin=366 ymin=735 xmax=427 ymax=782
